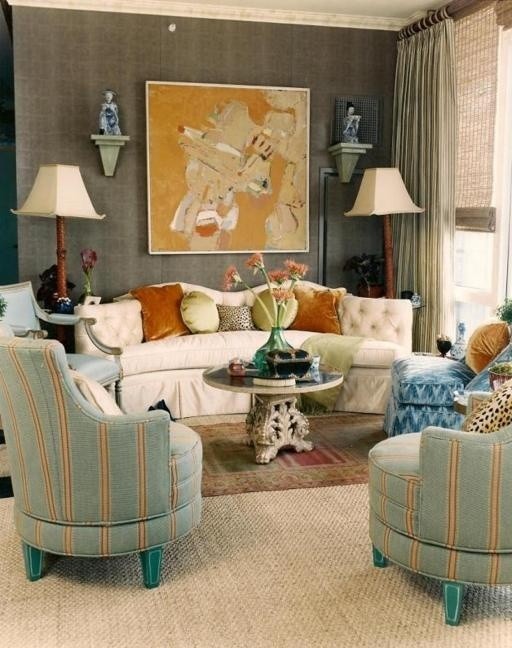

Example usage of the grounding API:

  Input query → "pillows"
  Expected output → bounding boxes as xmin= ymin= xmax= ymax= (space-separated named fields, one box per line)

xmin=461 ymin=378 xmax=512 ymax=434
xmin=128 ymin=283 xmax=343 ymax=342
xmin=69 ymin=368 xmax=125 ymax=416
xmin=465 ymin=322 xmax=512 ymax=375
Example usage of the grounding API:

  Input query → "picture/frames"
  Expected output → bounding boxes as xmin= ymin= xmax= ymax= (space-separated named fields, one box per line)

xmin=144 ymin=79 xmax=311 ymax=256
xmin=319 ymin=167 xmax=384 ymax=298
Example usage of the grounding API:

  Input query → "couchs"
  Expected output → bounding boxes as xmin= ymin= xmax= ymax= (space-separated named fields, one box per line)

xmin=73 ymin=279 xmax=414 ymax=421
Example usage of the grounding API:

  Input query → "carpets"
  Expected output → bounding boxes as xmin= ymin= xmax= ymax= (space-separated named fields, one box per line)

xmin=188 ymin=414 xmax=389 ymax=498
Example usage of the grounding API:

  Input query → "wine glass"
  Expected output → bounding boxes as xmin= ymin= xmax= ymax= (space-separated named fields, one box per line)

xmin=437 ymin=340 xmax=451 ymax=358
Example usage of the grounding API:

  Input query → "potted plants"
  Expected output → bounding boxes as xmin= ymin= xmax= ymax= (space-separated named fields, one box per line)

xmin=341 ymin=252 xmax=385 ymax=297
xmin=489 ymin=361 xmax=512 ymax=391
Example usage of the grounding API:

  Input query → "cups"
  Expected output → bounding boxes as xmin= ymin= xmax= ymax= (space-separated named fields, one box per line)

xmin=312 ymin=355 xmax=320 ymax=367
xmin=401 ymin=291 xmax=413 ymax=299
xmin=411 ymin=295 xmax=420 ymax=306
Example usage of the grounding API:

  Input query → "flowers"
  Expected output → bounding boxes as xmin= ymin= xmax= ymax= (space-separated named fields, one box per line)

xmin=80 ymin=247 xmax=97 ymax=296
xmin=223 ymin=252 xmax=311 ymax=327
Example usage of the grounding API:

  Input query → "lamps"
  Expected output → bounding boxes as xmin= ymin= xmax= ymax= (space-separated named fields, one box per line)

xmin=11 ymin=163 xmax=107 ymax=346
xmin=344 ymin=167 xmax=425 ymax=299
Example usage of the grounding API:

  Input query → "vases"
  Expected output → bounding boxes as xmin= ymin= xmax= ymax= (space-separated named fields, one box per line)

xmin=252 ymin=327 xmax=299 ymax=379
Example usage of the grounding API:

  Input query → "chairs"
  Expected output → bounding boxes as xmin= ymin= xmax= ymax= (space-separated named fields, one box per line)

xmin=0 ymin=332 xmax=204 ymax=589
xmin=382 ymin=322 xmax=512 ymax=438
xmin=0 ymin=280 xmax=125 ymax=408
xmin=367 ymin=391 xmax=512 ymax=626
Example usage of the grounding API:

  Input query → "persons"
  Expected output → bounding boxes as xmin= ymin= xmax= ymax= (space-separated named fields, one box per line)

xmin=341 ymin=101 xmax=363 ymax=144
xmin=98 ymin=87 xmax=122 ymax=135
